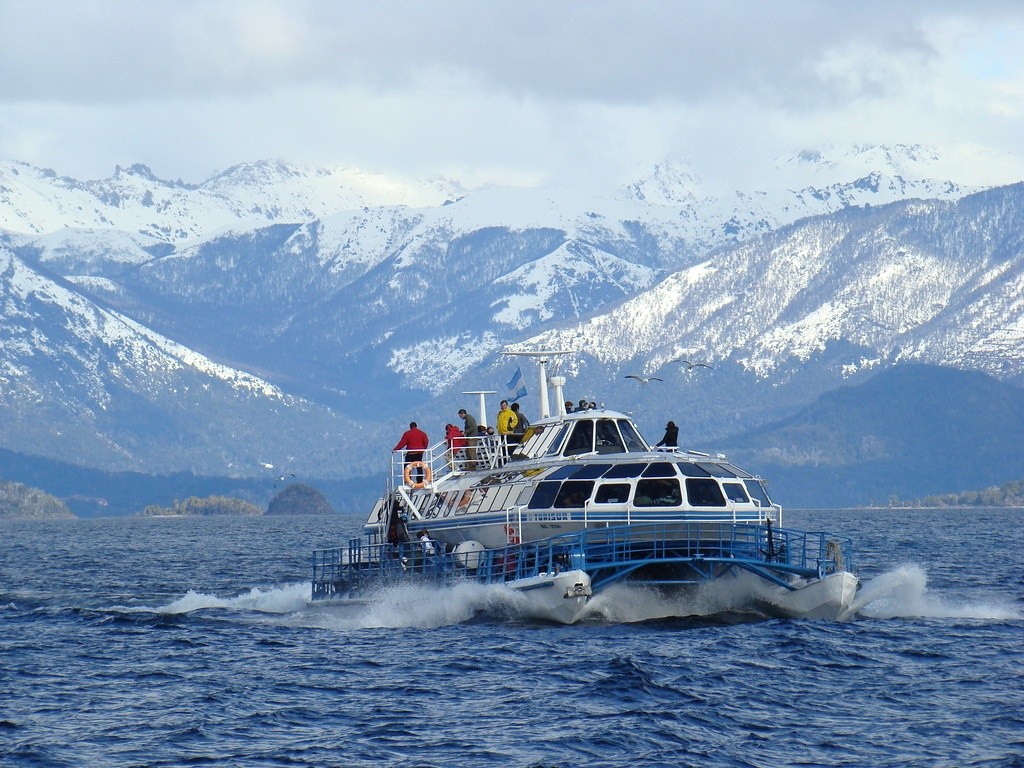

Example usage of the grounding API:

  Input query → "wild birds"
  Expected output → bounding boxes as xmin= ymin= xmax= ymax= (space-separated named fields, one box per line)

xmin=673 ymin=359 xmax=714 ymax=371
xmin=273 ymin=473 xmax=295 ymax=488
xmin=624 ymin=375 xmax=663 ymax=384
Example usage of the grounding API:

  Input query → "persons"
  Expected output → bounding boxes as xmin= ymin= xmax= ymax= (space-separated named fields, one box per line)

xmin=393 ymin=422 xmax=428 ymax=483
xmin=378 ymin=493 xmax=403 ymax=547
xmin=498 ymin=525 xmax=520 ymax=582
xmin=656 ymin=421 xmax=679 ymax=452
xmin=601 ymin=427 xmax=617 ymax=446
xmin=417 ymin=529 xmax=441 ymax=557
xmin=444 ymin=400 xmax=596 ymax=471
xmin=458 ymin=409 xmax=478 ymax=470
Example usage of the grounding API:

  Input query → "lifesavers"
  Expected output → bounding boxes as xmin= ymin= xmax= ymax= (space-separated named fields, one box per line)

xmin=445 ymin=490 xmax=471 ymax=506
xmin=404 ymin=461 xmax=431 ymax=488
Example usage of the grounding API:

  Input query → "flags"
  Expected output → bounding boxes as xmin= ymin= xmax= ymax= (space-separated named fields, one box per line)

xmin=506 ymin=366 xmax=528 ymax=402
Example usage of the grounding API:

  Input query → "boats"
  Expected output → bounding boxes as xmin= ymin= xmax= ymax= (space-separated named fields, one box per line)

xmin=303 ymin=348 xmax=865 ymax=625
